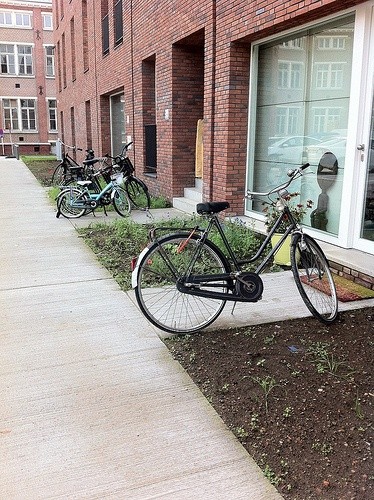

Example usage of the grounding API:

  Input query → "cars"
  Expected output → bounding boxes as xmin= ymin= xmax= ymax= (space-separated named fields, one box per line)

xmin=266 ymin=128 xmax=374 ymax=183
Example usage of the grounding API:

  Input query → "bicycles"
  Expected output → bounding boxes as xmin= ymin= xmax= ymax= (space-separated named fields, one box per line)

xmin=60 ymin=142 xmax=151 ymax=212
xmin=51 ymin=138 xmax=110 ymax=190
xmin=54 ymin=164 xmax=131 ymax=219
xmin=130 ymin=163 xmax=339 ymax=334
